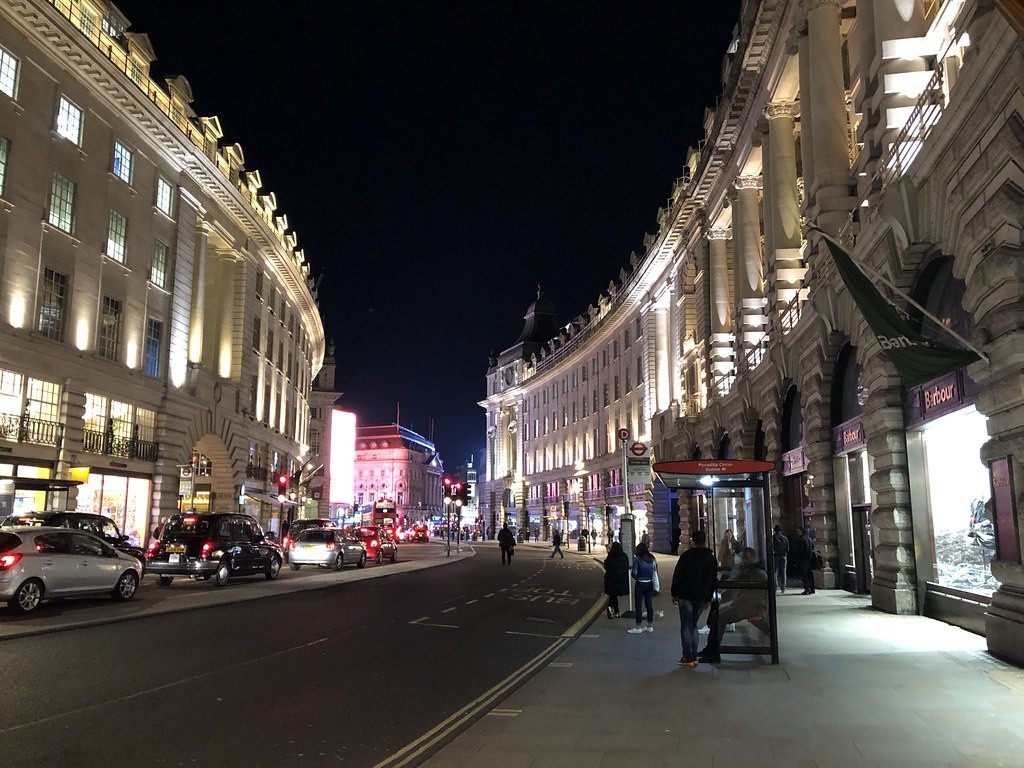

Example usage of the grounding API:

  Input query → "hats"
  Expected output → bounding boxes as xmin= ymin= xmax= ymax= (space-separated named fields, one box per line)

xmin=774 ymin=525 xmax=781 ymax=531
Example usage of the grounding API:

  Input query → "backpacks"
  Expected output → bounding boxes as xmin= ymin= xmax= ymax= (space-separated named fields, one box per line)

xmin=803 ymin=535 xmax=824 ymax=571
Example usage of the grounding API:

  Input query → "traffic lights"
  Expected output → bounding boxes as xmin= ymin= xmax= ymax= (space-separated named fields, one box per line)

xmin=455 ymin=482 xmax=463 ymax=499
xmin=445 ymin=479 xmax=452 ymax=497
xmin=461 ymin=481 xmax=472 ymax=506
xmin=278 ymin=476 xmax=286 ymax=497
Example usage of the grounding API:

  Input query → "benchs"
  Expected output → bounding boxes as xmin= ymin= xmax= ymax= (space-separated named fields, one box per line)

xmin=748 ymin=616 xmax=763 ymax=622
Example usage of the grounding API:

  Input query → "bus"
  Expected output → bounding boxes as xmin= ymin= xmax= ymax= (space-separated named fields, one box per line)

xmin=372 ymin=498 xmax=397 ymax=531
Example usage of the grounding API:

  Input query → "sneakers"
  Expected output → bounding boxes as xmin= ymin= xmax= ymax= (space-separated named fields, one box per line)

xmin=677 ymin=656 xmax=694 ymax=666
xmin=725 ymin=623 xmax=735 ymax=631
xmin=698 ymin=625 xmax=710 ymax=634
xmin=627 ymin=628 xmax=642 ymax=634
xmin=643 ymin=626 xmax=653 ymax=633
xmin=693 ymin=656 xmax=698 ymax=664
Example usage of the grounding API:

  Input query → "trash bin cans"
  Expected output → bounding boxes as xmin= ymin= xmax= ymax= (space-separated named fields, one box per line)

xmin=578 ymin=536 xmax=587 ymax=551
xmin=517 ymin=535 xmax=524 ymax=543
xmin=265 ymin=531 xmax=274 ymax=542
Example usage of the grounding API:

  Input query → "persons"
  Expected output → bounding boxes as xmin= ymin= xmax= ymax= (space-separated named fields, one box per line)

xmin=591 ymin=529 xmax=597 ymax=547
xmin=434 ymin=528 xmax=477 ymax=541
xmin=627 ymin=542 xmax=658 ymax=634
xmin=282 ymin=519 xmax=290 ymax=535
xmin=549 ymin=531 xmax=564 ymax=559
xmin=580 ymin=527 xmax=589 ymax=543
xmin=518 ymin=527 xmax=540 ymax=543
xmin=718 ymin=529 xmax=743 ymax=571
xmin=697 ymin=548 xmax=768 ymax=664
xmin=642 ymin=530 xmax=650 ymax=550
xmin=606 ymin=528 xmax=614 ymax=544
xmin=772 ymin=525 xmax=789 ymax=594
xmin=497 ymin=522 xmax=516 ymax=567
xmin=671 ymin=530 xmax=717 ymax=667
xmin=793 ymin=527 xmax=815 ymax=595
xmin=486 ymin=527 xmax=490 ymax=540
xmin=603 ymin=541 xmax=630 ymax=620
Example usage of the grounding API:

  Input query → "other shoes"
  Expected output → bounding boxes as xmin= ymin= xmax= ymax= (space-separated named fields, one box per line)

xmin=698 ymin=651 xmax=704 ymax=657
xmin=781 ymin=588 xmax=785 ymax=593
xmin=549 ymin=555 xmax=554 ymax=558
xmin=613 ymin=614 xmax=620 ymax=618
xmin=606 ymin=606 xmax=613 ymax=620
xmin=801 ymin=590 xmax=815 ymax=595
xmin=560 ymin=556 xmax=564 ymax=559
xmin=698 ymin=657 xmax=720 ymax=664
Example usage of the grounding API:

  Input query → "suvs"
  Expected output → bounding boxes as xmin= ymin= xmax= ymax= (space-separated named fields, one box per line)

xmin=281 ymin=517 xmax=336 ymax=563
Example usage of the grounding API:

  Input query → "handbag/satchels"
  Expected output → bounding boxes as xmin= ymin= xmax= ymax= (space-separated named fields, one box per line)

xmin=652 ymin=558 xmax=660 ymax=592
xmin=511 ymin=548 xmax=514 ymax=555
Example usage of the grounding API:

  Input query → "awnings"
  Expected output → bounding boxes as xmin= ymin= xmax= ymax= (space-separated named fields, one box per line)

xmin=246 ymin=491 xmax=300 ymax=508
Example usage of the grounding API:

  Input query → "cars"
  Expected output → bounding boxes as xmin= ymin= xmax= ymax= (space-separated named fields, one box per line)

xmin=0 ymin=511 xmax=149 ymax=579
xmin=384 ymin=522 xmax=430 ymax=544
xmin=354 ymin=526 xmax=398 ymax=564
xmin=289 ymin=526 xmax=367 ymax=570
xmin=0 ymin=524 xmax=144 ymax=616
xmin=146 ymin=509 xmax=283 ymax=588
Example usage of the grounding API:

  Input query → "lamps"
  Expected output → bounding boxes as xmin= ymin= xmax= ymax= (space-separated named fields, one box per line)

xmin=278 ymin=491 xmax=285 ymax=504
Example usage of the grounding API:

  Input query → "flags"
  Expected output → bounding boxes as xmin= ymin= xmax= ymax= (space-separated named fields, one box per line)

xmin=822 ymin=234 xmax=991 ymax=387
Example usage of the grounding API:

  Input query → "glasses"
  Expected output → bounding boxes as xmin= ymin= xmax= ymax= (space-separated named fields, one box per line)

xmin=689 ymin=538 xmax=693 ymax=542
